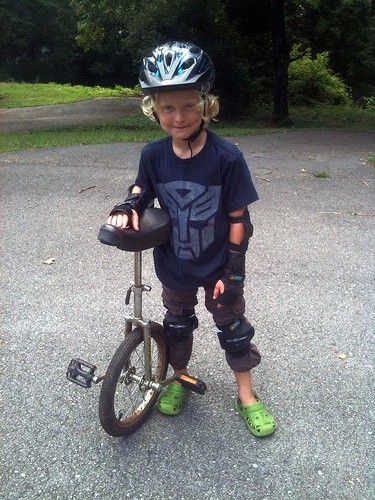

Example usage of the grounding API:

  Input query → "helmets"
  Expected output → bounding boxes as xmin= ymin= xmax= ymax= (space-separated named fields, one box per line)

xmin=138 ymin=41 xmax=214 ymax=95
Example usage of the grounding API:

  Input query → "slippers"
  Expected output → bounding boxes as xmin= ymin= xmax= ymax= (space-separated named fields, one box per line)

xmin=235 ymin=392 xmax=276 ymax=436
xmin=157 ymin=380 xmax=189 ymax=415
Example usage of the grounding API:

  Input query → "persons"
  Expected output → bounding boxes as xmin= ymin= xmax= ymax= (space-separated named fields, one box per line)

xmin=106 ymin=40 xmax=277 ymax=437
